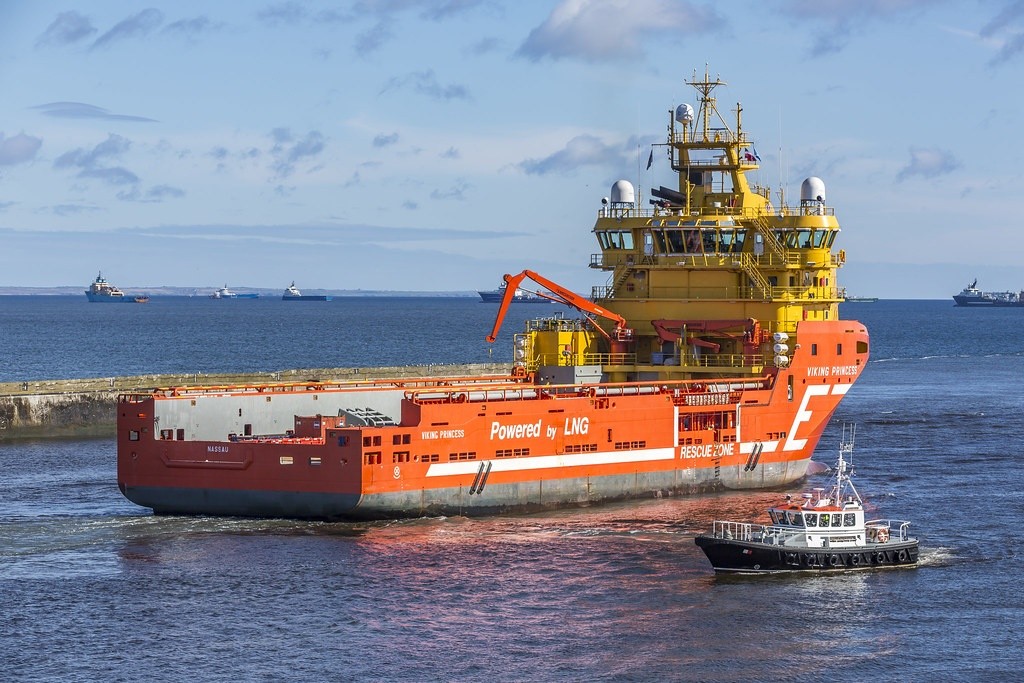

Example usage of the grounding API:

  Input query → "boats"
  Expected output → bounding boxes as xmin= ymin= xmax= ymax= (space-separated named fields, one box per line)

xmin=219 ymin=284 xmax=259 ymax=298
xmin=85 ymin=269 xmax=151 ymax=303
xmin=477 ymin=282 xmax=556 ymax=303
xmin=282 ymin=281 xmax=334 ymax=302
xmin=850 ymin=297 xmax=880 ymax=303
xmin=214 ymin=291 xmax=222 ymax=299
xmin=113 ymin=61 xmax=870 ymax=519
xmin=953 ymin=277 xmax=1024 ymax=307
xmin=693 ymin=423 xmax=919 ymax=571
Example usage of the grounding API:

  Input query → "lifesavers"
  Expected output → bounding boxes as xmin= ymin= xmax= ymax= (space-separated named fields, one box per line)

xmin=878 ymin=529 xmax=889 ymax=542
xmin=877 ymin=551 xmax=885 ymax=563
xmin=806 ymin=554 xmax=816 ymax=566
xmin=899 ymin=550 xmax=907 ymax=561
xmin=852 ymin=553 xmax=860 ymax=565
xmin=785 ymin=553 xmax=795 ymax=564
